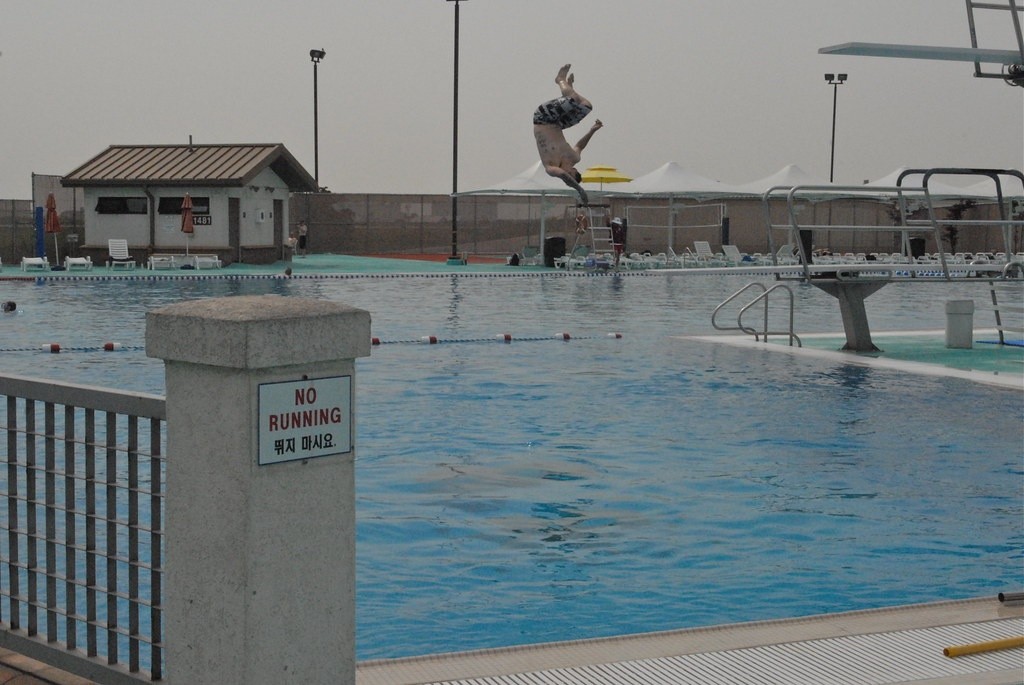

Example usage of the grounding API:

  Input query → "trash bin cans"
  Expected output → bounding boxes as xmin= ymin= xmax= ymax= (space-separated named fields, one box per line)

xmin=905 ymin=237 xmax=925 ymax=259
xmin=544 ymin=237 xmax=566 ymax=268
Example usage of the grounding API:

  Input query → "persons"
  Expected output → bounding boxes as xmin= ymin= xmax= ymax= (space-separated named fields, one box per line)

xmin=606 ymin=217 xmax=624 ymax=265
xmin=288 ymin=233 xmax=297 ymax=256
xmin=298 ymin=220 xmax=307 ymax=258
xmin=533 ymin=64 xmax=602 ymax=207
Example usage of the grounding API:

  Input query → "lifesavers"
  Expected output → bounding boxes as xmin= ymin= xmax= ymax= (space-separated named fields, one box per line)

xmin=575 ymin=214 xmax=587 ymax=234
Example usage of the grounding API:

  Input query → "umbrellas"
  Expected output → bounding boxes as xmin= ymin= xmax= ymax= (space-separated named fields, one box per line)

xmin=46 ymin=192 xmax=65 ymax=271
xmin=181 ymin=192 xmax=194 ymax=269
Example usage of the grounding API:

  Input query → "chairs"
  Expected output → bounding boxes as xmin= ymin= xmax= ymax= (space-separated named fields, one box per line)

xmin=105 ymin=239 xmax=136 ymax=269
xmin=65 ymin=256 xmax=94 ymax=271
xmin=145 ymin=256 xmax=176 ymax=271
xmin=21 ymin=256 xmax=52 ymax=273
xmin=507 ymin=240 xmax=1024 ymax=270
xmin=193 ymin=256 xmax=222 ymax=270
xmin=288 ymin=238 xmax=298 ymax=255
